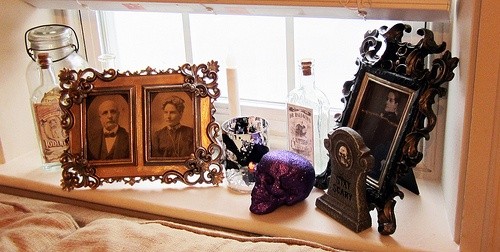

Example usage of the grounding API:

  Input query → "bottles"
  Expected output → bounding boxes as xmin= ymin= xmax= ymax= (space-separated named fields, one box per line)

xmin=25 ymin=27 xmax=93 ymax=103
xmin=287 ymin=60 xmax=331 ymax=176
xmin=98 ymin=53 xmax=118 ymax=72
xmin=29 ymin=52 xmax=72 ymax=172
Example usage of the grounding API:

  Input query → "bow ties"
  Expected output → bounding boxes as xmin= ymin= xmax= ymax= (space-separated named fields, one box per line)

xmin=104 ymin=132 xmax=117 ymax=138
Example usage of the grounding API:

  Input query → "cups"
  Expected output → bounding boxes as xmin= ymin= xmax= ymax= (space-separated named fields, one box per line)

xmin=221 ymin=116 xmax=269 ymax=194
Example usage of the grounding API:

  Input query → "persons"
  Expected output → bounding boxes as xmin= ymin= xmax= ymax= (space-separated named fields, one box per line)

xmin=368 ymin=89 xmax=401 ymax=181
xmin=88 ymin=100 xmax=130 ymax=160
xmin=151 ymin=95 xmax=194 ymax=157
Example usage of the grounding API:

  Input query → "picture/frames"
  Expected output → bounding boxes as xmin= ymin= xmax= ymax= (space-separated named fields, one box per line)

xmin=313 ymin=22 xmax=459 ymax=237
xmin=58 ymin=59 xmax=225 ymax=192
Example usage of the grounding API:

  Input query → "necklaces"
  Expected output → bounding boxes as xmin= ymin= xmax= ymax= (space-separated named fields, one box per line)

xmin=167 ymin=123 xmax=180 ymax=132
